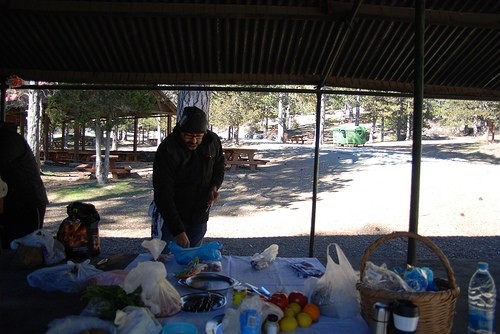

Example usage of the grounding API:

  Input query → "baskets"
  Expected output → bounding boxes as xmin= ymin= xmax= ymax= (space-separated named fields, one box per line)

xmin=356 ymin=232 xmax=460 ymax=334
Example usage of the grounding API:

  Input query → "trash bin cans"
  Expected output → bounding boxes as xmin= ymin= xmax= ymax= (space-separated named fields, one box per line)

xmin=333 ymin=126 xmax=365 ymax=146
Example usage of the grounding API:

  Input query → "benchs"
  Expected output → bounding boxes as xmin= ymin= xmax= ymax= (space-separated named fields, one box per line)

xmin=38 ymin=136 xmax=308 ymax=179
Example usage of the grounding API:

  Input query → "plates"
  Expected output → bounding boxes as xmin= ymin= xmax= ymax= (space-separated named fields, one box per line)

xmin=178 ymin=272 xmax=238 ymax=295
xmin=178 ymin=291 xmax=227 ymax=315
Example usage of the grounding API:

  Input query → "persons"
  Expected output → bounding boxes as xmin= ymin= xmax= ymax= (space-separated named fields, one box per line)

xmin=148 ymin=106 xmax=226 ymax=252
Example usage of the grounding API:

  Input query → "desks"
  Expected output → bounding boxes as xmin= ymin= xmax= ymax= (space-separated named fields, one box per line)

xmin=79 ymin=152 xmax=93 ymax=162
xmin=91 ymin=155 xmax=118 ymax=173
xmin=222 ymin=148 xmax=257 ymax=173
xmin=49 ymin=149 xmax=68 ymax=162
xmin=292 ymin=136 xmax=304 ymax=145
xmin=120 ymin=152 xmax=137 ymax=161
xmin=80 ymin=253 xmax=369 ymax=334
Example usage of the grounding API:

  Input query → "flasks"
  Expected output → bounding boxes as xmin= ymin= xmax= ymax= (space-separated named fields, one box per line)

xmin=370 ymin=302 xmax=389 ymax=334
xmin=262 ymin=314 xmax=279 ymax=334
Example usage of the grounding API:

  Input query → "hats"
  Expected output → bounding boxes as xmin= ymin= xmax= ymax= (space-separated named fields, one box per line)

xmin=179 ymin=106 xmax=206 ymax=133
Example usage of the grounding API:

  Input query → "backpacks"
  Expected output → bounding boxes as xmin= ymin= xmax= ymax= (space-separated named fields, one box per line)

xmin=57 ymin=202 xmax=100 ymax=258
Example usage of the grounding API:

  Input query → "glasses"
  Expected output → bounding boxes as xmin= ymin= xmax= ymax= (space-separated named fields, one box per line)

xmin=181 ymin=133 xmax=204 ymax=139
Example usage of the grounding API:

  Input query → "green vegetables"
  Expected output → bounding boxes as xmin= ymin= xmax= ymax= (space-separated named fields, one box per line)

xmin=80 ymin=283 xmax=152 ymax=320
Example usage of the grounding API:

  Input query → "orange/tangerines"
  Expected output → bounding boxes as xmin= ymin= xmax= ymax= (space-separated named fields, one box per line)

xmin=302 ymin=303 xmax=320 ymax=321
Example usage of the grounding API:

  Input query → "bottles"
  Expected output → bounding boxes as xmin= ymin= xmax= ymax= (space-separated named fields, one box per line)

xmin=467 ymin=262 xmax=495 ymax=334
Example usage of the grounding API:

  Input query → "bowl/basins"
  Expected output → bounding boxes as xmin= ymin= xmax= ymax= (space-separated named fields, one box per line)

xmin=161 ymin=322 xmax=199 ymax=334
xmin=205 ymin=313 xmax=225 ymax=334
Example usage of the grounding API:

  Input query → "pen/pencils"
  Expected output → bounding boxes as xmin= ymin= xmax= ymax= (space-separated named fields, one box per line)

xmin=244 ymin=283 xmax=270 ymax=300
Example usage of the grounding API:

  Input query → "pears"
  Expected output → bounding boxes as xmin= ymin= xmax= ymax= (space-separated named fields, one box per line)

xmin=279 ymin=303 xmax=312 ymax=331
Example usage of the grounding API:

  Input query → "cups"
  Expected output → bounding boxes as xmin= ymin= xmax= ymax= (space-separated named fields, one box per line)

xmin=389 ymin=299 xmax=419 ymax=334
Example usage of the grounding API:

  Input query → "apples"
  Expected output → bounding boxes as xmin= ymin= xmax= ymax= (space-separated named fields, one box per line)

xmin=288 ymin=290 xmax=308 ymax=309
xmin=270 ymin=292 xmax=288 ymax=309
